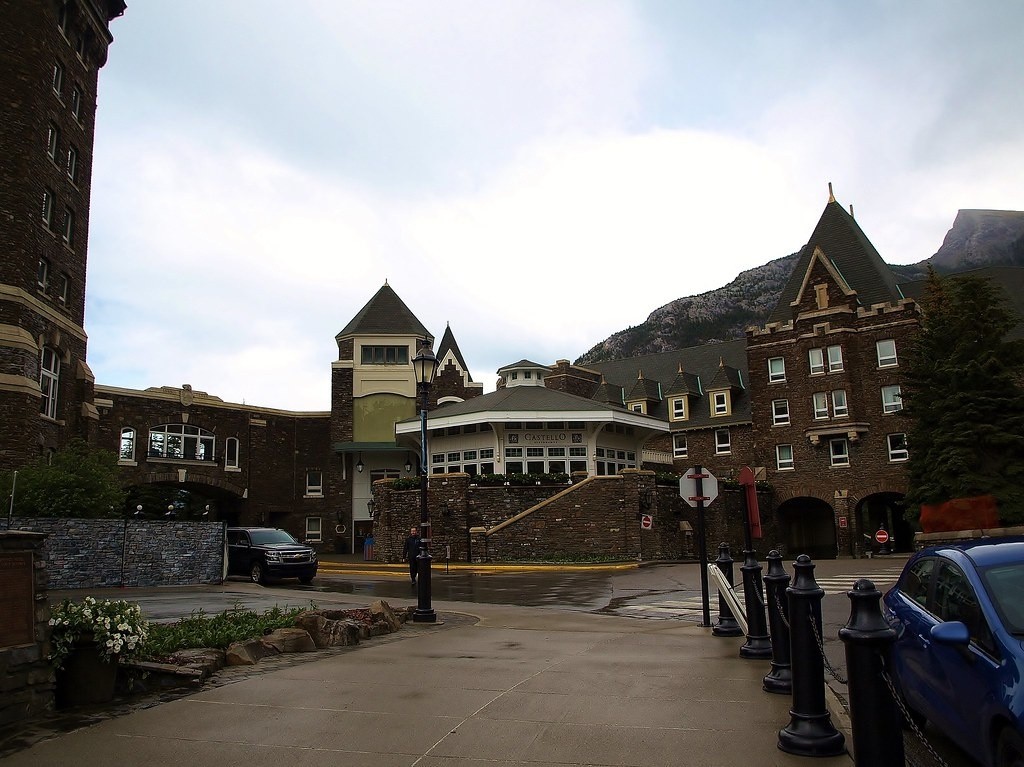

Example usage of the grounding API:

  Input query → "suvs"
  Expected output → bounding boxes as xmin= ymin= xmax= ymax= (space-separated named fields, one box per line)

xmin=225 ymin=527 xmax=317 ymax=586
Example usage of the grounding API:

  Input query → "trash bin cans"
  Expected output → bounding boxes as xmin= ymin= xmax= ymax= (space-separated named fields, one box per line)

xmin=364 ymin=538 xmax=374 ymax=560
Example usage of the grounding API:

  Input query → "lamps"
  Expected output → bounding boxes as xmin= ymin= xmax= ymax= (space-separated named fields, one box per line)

xmin=261 ymin=509 xmax=269 ymax=524
xmin=367 ymin=498 xmax=381 ymax=517
xmin=336 ymin=509 xmax=345 ymax=525
xmin=439 ymin=500 xmax=452 ymax=517
xmin=356 ymin=451 xmax=365 ymax=474
xmin=404 ymin=452 xmax=413 ymax=473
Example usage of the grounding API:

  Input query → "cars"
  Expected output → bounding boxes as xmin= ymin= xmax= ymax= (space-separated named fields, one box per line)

xmin=879 ymin=535 xmax=1024 ymax=767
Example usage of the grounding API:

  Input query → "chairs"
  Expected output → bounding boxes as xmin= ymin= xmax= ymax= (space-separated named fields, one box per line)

xmin=943 ymin=575 xmax=979 ymax=636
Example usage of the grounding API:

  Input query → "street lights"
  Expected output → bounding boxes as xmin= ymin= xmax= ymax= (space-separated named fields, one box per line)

xmin=406 ymin=332 xmax=446 ymax=625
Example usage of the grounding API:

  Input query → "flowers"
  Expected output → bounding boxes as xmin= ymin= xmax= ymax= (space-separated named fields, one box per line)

xmin=49 ymin=597 xmax=151 ymax=696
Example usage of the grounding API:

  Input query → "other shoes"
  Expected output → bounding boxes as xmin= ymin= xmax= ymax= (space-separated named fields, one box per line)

xmin=412 ymin=580 xmax=416 ymax=584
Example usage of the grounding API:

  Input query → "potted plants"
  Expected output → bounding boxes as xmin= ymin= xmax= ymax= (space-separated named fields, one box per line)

xmin=392 ymin=476 xmax=422 ymax=490
xmin=473 ymin=473 xmax=570 ymax=486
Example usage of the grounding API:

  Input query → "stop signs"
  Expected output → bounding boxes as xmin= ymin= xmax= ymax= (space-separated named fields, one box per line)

xmin=640 ymin=514 xmax=653 ymax=530
xmin=874 ymin=531 xmax=889 ymax=543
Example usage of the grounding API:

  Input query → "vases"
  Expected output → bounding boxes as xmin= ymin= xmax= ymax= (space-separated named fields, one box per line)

xmin=64 ymin=648 xmax=117 ymax=704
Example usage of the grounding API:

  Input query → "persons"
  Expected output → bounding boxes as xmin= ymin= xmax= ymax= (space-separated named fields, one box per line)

xmin=403 ymin=527 xmax=421 ymax=584
